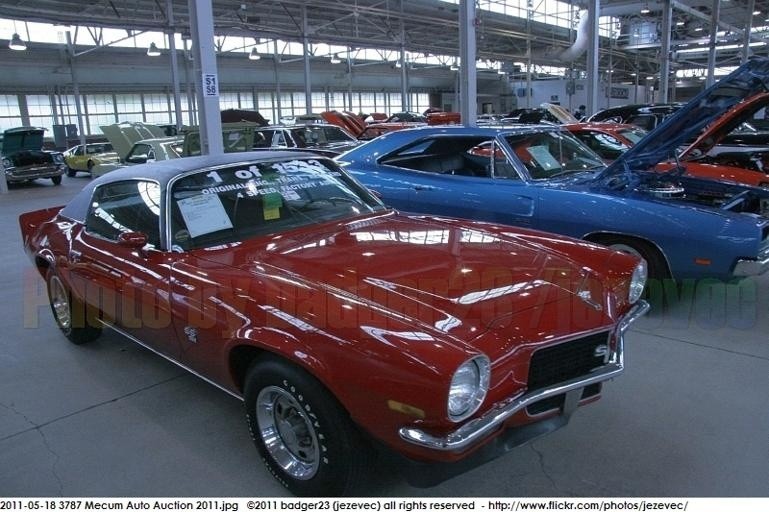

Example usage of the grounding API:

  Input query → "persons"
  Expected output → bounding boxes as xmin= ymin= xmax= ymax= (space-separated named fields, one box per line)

xmin=574 ymin=104 xmax=586 ymax=122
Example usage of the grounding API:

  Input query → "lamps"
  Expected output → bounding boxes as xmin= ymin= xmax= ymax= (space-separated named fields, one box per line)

xmin=9 ymin=15 xmax=461 ymax=74
xmin=752 ymin=2 xmax=761 ymax=16
xmin=640 ymin=1 xmax=652 ymax=14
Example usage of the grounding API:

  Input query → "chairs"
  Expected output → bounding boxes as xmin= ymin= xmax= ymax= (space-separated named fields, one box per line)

xmin=418 ymin=148 xmax=516 ymax=178
